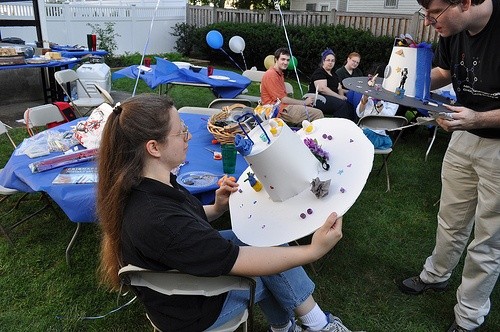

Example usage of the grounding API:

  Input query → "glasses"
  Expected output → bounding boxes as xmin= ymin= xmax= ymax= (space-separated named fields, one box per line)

xmin=324 ymin=59 xmax=335 ymax=62
xmin=416 ymin=2 xmax=455 ymax=26
xmin=170 ymin=122 xmax=192 ymax=137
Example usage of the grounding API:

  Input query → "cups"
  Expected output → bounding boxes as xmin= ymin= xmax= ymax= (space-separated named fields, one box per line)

xmin=144 ymin=58 xmax=150 ymax=67
xmin=208 ymin=66 xmax=213 ymax=75
xmin=222 ymin=144 xmax=237 ymax=174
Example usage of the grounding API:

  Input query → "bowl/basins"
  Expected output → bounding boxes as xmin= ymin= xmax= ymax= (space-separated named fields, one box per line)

xmin=192 ymin=68 xmax=202 ymax=73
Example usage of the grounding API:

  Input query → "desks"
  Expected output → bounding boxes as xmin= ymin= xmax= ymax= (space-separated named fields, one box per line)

xmin=0 ymin=111 xmax=249 ymax=272
xmin=113 ymin=61 xmax=251 ymax=100
xmin=28 ymin=42 xmax=108 ymax=101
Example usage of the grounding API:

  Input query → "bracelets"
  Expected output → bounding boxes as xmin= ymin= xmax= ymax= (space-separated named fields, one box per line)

xmin=375 ymin=104 xmax=383 ymax=107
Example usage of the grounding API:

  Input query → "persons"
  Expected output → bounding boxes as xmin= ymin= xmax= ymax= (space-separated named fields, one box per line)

xmin=399 ymin=0 xmax=500 ymax=332
xmin=260 ymin=47 xmax=324 ymax=126
xmin=336 ymin=52 xmax=364 ymax=97
xmin=356 ymin=95 xmax=399 ymax=150
xmin=96 ymin=92 xmax=352 ymax=332
xmin=308 ymin=49 xmax=355 ymax=121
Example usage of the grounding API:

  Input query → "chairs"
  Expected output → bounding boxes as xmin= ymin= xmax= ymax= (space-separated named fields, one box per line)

xmin=0 ymin=69 xmax=407 ymax=332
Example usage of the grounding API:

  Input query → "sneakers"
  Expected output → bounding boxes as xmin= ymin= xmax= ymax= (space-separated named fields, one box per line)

xmin=446 ymin=322 xmax=473 ymax=332
xmin=401 ymin=274 xmax=451 ymax=295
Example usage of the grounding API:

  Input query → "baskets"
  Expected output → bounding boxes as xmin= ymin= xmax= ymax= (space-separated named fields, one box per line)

xmin=207 ymin=104 xmax=257 ymax=149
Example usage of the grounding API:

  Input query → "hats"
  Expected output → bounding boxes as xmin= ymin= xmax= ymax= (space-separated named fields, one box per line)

xmin=228 ymin=112 xmax=376 ymax=246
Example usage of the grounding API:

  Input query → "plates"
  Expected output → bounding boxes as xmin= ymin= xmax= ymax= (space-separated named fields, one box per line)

xmin=176 ymin=171 xmax=218 ymax=187
xmin=209 ymin=75 xmax=230 ymax=80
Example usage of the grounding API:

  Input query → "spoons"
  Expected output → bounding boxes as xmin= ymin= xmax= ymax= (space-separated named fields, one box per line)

xmin=192 ymin=175 xmax=223 ymax=179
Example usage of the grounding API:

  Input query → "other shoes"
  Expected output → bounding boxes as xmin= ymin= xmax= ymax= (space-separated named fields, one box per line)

xmin=286 ymin=312 xmax=353 ymax=332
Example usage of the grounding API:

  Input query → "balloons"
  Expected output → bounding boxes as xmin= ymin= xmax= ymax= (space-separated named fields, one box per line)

xmin=229 ymin=36 xmax=245 ymax=53
xmin=206 ymin=30 xmax=224 ymax=49
xmin=286 ymin=55 xmax=298 ymax=70
xmin=264 ymin=55 xmax=275 ymax=69
